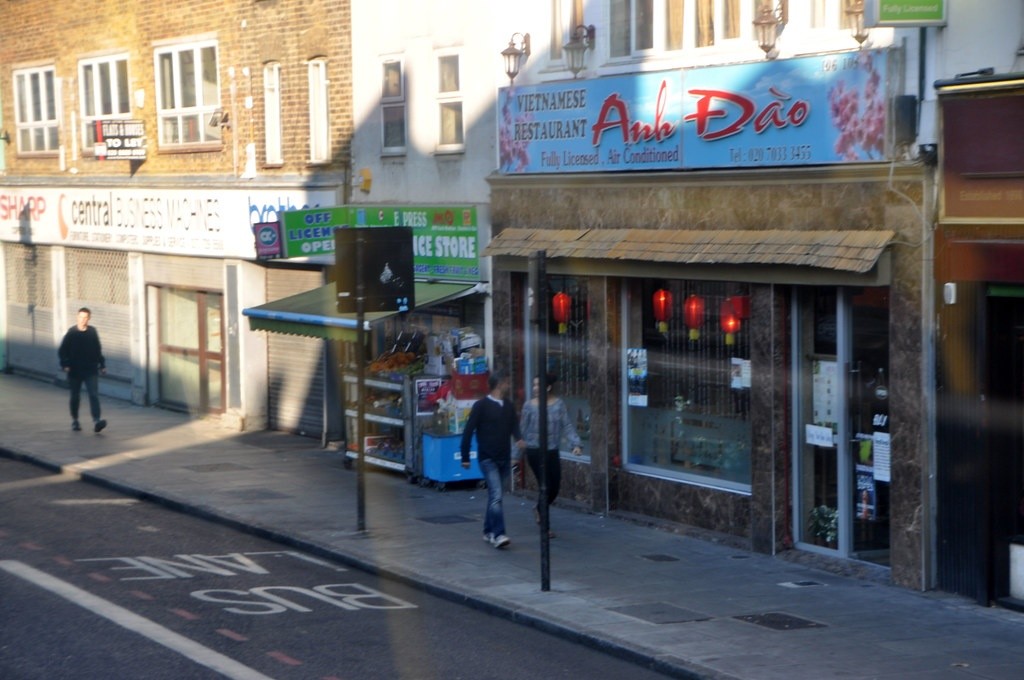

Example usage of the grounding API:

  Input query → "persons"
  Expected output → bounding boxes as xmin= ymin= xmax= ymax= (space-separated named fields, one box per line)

xmin=58 ymin=309 xmax=109 ymax=433
xmin=461 ymin=367 xmax=526 ymax=548
xmin=519 ymin=376 xmax=585 ymax=539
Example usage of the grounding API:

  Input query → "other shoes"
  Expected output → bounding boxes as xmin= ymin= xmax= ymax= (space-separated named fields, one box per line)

xmin=494 ymin=535 xmax=510 ymax=548
xmin=95 ymin=419 xmax=107 ymax=431
xmin=483 ymin=531 xmax=495 ymax=544
xmin=72 ymin=421 xmax=80 ymax=430
xmin=533 ymin=503 xmax=542 ymax=523
xmin=548 ymin=531 xmax=555 ymax=538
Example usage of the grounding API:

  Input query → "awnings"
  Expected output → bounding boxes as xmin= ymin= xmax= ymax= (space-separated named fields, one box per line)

xmin=244 ymin=277 xmax=483 ymax=346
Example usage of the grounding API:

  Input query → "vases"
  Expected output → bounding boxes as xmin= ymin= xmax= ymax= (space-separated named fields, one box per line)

xmin=813 ymin=531 xmax=829 ymax=548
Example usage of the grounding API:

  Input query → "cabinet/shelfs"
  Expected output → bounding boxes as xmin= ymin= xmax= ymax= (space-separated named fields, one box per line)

xmin=337 ymin=360 xmax=447 ymax=487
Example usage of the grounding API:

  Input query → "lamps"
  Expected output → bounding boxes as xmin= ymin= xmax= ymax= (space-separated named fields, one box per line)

xmin=501 ymin=32 xmax=530 ymax=84
xmin=208 ymin=107 xmax=229 ymax=129
xmin=0 ymin=129 xmax=9 ymax=139
xmin=845 ymin=0 xmax=869 ymax=50
xmin=751 ymin=0 xmax=789 ymax=59
xmin=562 ymin=25 xmax=596 ymax=79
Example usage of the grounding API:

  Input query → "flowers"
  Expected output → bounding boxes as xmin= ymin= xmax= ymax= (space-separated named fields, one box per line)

xmin=806 ymin=503 xmax=837 ymax=544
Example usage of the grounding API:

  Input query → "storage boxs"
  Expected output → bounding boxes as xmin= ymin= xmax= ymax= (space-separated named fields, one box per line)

xmin=423 ymin=326 xmax=490 ymax=436
